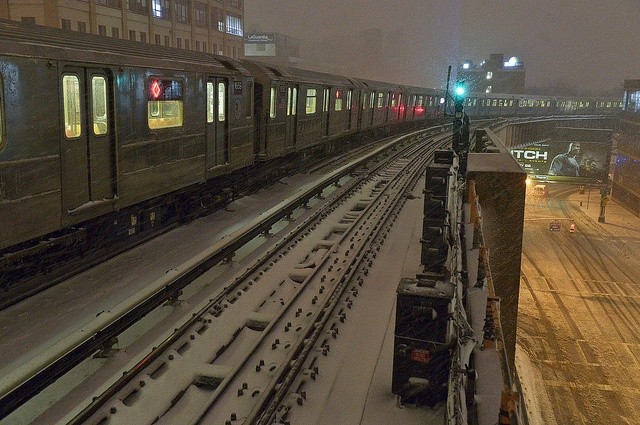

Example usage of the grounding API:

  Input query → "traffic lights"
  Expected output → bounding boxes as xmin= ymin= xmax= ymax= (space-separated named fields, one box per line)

xmin=453 ymin=80 xmax=465 ymax=149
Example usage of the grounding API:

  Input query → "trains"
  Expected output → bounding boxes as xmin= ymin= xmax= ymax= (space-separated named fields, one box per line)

xmin=0 ymin=18 xmax=623 ymax=292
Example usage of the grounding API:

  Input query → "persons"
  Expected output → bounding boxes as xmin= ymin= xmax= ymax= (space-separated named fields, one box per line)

xmin=548 ymin=142 xmax=580 ymax=177
xmin=579 ymin=157 xmax=598 ymax=177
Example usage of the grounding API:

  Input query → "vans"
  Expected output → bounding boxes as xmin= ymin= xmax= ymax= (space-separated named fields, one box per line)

xmin=532 ymin=185 xmax=546 ymax=197
